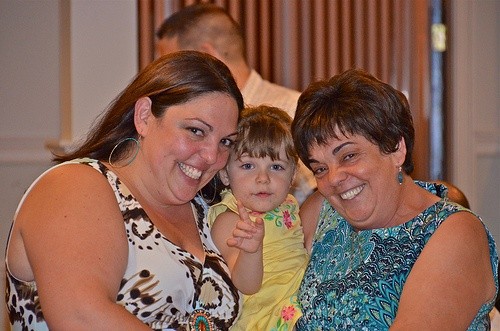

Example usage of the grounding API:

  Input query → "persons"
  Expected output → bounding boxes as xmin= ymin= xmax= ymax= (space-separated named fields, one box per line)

xmin=291 ymin=67 xmax=500 ymax=331
xmin=4 ymin=50 xmax=244 ymax=331
xmin=156 ymin=3 xmax=319 ymax=207
xmin=208 ymin=103 xmax=310 ymax=331
